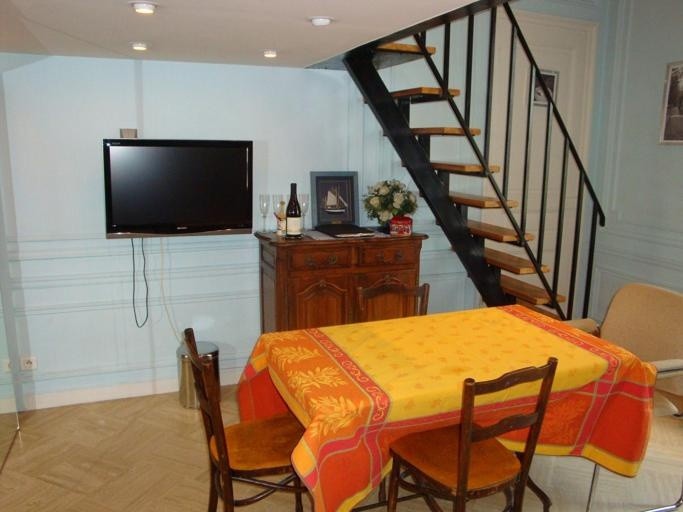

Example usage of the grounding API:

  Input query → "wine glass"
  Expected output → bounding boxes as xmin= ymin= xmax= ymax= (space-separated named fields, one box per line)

xmin=258 ymin=194 xmax=310 ymax=235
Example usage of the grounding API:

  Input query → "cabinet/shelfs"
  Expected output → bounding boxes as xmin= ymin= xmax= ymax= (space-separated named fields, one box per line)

xmin=251 ymin=224 xmax=429 ymax=334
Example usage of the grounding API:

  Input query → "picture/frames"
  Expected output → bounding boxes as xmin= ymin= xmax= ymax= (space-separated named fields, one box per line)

xmin=306 ymin=169 xmax=361 ymax=230
xmin=527 ymin=66 xmax=560 ymax=109
xmin=655 ymin=60 xmax=682 ymax=146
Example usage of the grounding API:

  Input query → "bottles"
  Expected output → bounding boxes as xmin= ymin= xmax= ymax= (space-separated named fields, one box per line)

xmin=285 ymin=183 xmax=300 ymax=238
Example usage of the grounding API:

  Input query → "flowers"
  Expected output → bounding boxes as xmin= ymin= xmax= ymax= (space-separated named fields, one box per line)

xmin=361 ymin=177 xmax=419 ymax=230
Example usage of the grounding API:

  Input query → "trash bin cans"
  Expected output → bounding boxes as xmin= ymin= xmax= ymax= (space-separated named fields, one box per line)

xmin=176 ymin=341 xmax=219 ymax=409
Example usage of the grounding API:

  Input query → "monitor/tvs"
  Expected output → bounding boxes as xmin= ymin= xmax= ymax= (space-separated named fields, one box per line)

xmin=102 ymin=138 xmax=253 ymax=239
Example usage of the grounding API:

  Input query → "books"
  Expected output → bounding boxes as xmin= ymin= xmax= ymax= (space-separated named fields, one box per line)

xmin=314 ymin=224 xmax=374 ymax=238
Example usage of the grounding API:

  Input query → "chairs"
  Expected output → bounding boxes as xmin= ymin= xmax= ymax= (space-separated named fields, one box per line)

xmin=354 ymin=282 xmax=429 ymax=321
xmin=547 ymin=281 xmax=682 ymax=512
xmin=381 ymin=356 xmax=558 ymax=512
xmin=179 ymin=326 xmax=313 ymax=512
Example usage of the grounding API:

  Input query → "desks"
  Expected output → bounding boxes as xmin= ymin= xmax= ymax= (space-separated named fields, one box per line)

xmin=231 ymin=299 xmax=661 ymax=512
